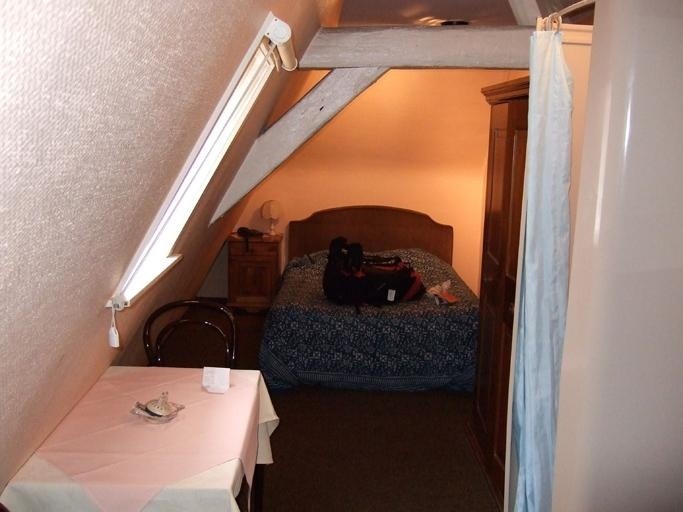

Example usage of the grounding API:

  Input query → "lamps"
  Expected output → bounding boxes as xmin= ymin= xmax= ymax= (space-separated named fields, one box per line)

xmin=259 ymin=198 xmax=284 ymax=236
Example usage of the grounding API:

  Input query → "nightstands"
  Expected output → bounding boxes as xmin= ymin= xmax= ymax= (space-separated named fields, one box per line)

xmin=225 ymin=230 xmax=283 ymax=316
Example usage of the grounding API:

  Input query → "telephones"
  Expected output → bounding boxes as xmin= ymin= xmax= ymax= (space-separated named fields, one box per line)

xmin=237 ymin=227 xmax=264 ymax=237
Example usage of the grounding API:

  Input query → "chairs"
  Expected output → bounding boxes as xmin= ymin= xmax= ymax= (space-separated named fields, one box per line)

xmin=138 ymin=298 xmax=238 ymax=365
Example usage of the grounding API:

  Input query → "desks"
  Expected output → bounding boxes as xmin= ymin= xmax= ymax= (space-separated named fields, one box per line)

xmin=2 ymin=366 xmax=262 ymax=512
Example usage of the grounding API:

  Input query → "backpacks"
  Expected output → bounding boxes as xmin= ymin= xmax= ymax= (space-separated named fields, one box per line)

xmin=322 ymin=236 xmax=426 ymax=305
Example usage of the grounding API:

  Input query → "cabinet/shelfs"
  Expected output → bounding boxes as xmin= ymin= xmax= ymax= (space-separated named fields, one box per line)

xmin=460 ymin=74 xmax=542 ymax=511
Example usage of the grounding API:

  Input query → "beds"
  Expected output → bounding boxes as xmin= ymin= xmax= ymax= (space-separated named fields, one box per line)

xmin=264 ymin=204 xmax=481 ymax=392
